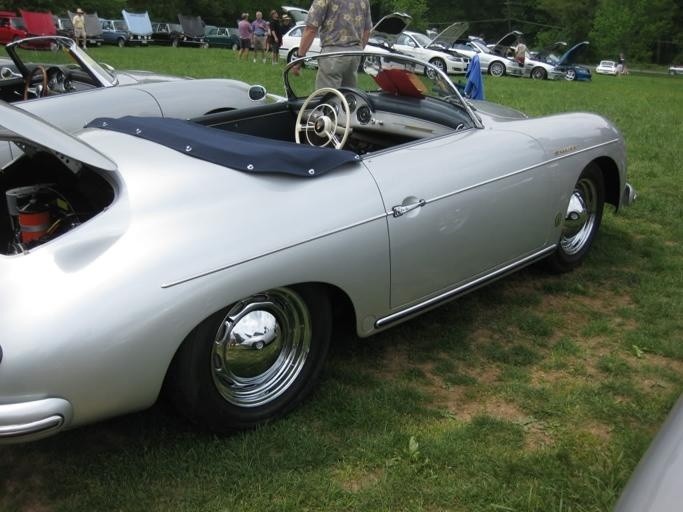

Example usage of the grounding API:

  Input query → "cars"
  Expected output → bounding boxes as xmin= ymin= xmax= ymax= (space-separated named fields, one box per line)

xmin=427 ymin=28 xmax=523 ymax=79
xmin=1 ymin=8 xmax=244 ymax=52
xmin=594 ymin=60 xmax=623 ymax=76
xmin=395 ymin=21 xmax=475 ymax=80
xmin=668 ymin=65 xmax=683 ymax=75
xmin=276 ymin=5 xmax=418 ymax=77
xmin=485 ymin=43 xmax=559 ymax=82
xmin=532 ymin=41 xmax=595 ymax=82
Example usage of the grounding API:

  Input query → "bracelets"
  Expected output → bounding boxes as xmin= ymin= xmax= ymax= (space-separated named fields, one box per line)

xmin=293 ymin=56 xmax=305 ymax=65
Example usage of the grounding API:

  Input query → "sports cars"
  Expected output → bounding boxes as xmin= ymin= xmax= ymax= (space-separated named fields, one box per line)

xmin=0 ymin=6 xmax=637 ymax=443
xmin=0 ymin=35 xmax=267 ymax=133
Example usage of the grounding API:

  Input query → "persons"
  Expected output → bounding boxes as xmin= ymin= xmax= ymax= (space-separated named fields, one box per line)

xmin=291 ymin=0 xmax=373 ymax=96
xmin=514 ymin=39 xmax=532 ymax=75
xmin=237 ymin=9 xmax=294 ymax=65
xmin=616 ymin=52 xmax=624 ymax=77
xmin=72 ymin=8 xmax=87 ymax=49
